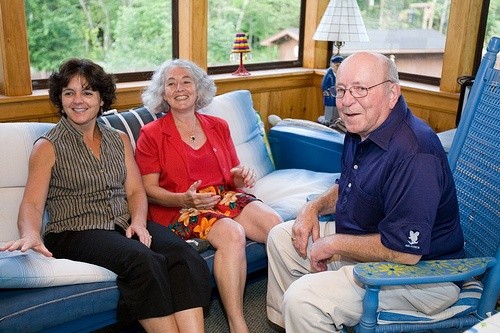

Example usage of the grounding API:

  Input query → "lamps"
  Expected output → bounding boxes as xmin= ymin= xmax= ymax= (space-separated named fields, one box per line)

xmin=311 ymin=0 xmax=370 ymax=125
xmin=231 ymin=34 xmax=253 ymax=76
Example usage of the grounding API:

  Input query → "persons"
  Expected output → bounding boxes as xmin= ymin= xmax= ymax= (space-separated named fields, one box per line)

xmin=133 ymin=59 xmax=286 ymax=333
xmin=265 ymin=51 xmax=466 ymax=333
xmin=322 ymin=56 xmax=344 ymax=123
xmin=0 ymin=58 xmax=213 ymax=333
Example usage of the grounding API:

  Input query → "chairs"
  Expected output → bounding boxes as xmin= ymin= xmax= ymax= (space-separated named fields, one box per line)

xmin=436 ymin=75 xmax=475 ymax=154
xmin=337 ymin=35 xmax=500 ymax=333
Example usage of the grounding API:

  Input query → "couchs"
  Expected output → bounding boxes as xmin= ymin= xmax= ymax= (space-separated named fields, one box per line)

xmin=1 ymin=91 xmax=352 ymax=333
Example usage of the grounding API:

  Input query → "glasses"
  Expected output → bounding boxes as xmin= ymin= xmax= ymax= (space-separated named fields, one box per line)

xmin=327 ymin=80 xmax=393 ymax=98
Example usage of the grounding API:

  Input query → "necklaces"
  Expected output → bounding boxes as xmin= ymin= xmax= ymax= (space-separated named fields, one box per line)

xmin=89 ymin=148 xmax=93 ymax=154
xmin=176 ymin=114 xmax=197 ymax=143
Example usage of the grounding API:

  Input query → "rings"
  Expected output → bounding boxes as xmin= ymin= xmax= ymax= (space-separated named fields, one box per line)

xmin=148 ymin=235 xmax=152 ymax=238
xmin=291 ymin=237 xmax=296 ymax=241
